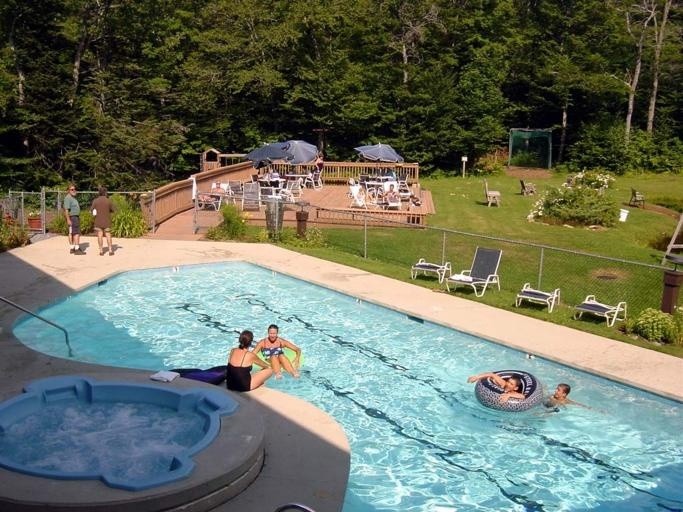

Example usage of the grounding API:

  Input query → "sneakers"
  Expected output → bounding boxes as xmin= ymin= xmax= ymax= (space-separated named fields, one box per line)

xmin=74 ymin=248 xmax=86 ymax=256
xmin=99 ymin=250 xmax=114 ymax=256
xmin=70 ymin=247 xmax=76 ymax=254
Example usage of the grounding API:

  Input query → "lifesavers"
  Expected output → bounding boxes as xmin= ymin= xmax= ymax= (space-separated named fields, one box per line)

xmin=474 ymin=369 xmax=546 ymax=413
xmin=253 ymin=346 xmax=305 ymax=370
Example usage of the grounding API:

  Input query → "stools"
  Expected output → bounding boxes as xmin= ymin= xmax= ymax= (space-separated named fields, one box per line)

xmin=410 ymin=257 xmax=452 ymax=285
xmin=514 ymin=282 xmax=627 ymax=328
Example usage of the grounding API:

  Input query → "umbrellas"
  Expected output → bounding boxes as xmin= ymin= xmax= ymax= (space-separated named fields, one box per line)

xmin=285 ymin=140 xmax=316 ymax=165
xmin=247 ymin=143 xmax=290 ymax=162
xmin=354 ymin=142 xmax=404 ymax=162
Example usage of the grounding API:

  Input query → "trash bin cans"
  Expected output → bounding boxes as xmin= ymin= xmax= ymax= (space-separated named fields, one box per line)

xmin=265 ymin=203 xmax=284 ymax=237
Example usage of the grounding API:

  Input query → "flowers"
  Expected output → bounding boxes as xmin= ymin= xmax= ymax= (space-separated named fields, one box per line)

xmin=27 ymin=206 xmax=40 ymax=217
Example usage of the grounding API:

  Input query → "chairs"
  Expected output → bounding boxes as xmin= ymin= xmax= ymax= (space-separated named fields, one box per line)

xmin=443 ymin=244 xmax=504 ymax=298
xmin=483 ymin=178 xmax=501 ymax=207
xmin=348 ymin=171 xmax=412 ymax=210
xmin=519 ymin=178 xmax=536 ymax=197
xmin=627 ymin=188 xmax=645 ymax=209
xmin=195 ymin=168 xmax=324 ymax=212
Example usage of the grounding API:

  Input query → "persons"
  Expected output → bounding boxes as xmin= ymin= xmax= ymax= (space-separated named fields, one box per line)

xmin=544 ymin=383 xmax=593 ymax=414
xmin=467 ymin=374 xmax=526 ymax=403
xmin=385 ymin=183 xmax=400 ymax=202
xmin=253 ymin=324 xmax=302 ymax=381
xmin=225 ymin=330 xmax=273 ymax=393
xmin=90 ymin=187 xmax=118 ymax=256
xmin=64 ymin=183 xmax=86 ymax=255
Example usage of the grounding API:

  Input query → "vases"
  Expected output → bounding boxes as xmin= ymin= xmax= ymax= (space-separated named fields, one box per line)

xmin=26 ymin=216 xmax=41 ymax=229
